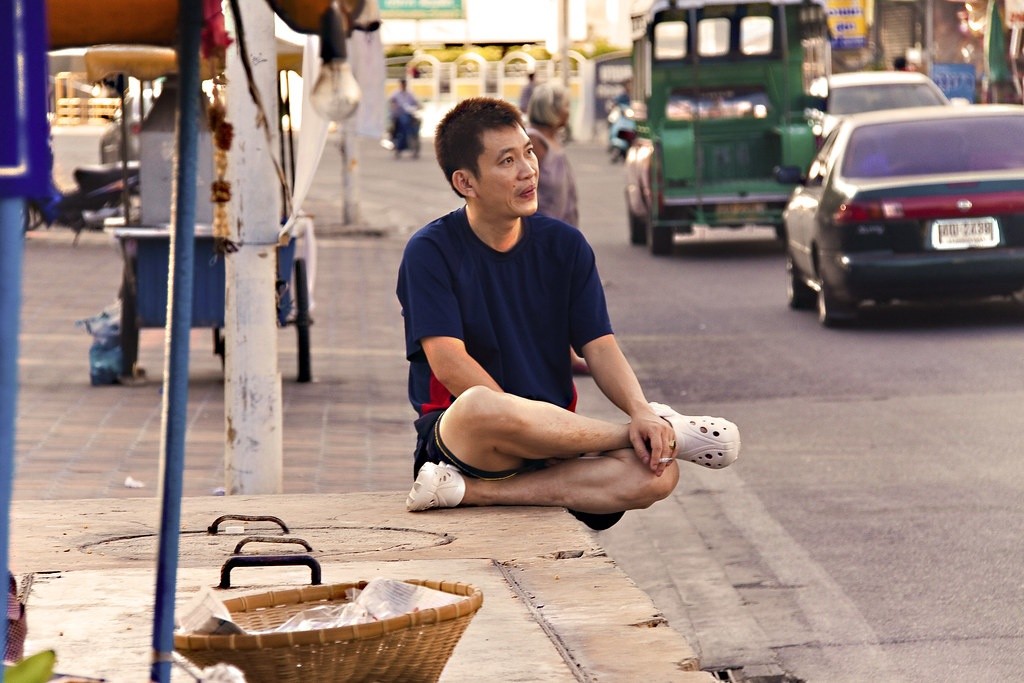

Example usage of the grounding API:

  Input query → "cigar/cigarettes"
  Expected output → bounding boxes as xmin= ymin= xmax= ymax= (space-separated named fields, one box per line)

xmin=659 ymin=457 xmax=672 ymax=463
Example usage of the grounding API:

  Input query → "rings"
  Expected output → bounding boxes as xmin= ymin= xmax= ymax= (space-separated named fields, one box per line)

xmin=668 ymin=440 xmax=676 ymax=449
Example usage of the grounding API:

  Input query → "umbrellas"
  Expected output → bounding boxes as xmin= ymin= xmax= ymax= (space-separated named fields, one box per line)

xmin=983 ymin=0 xmax=1012 ymax=104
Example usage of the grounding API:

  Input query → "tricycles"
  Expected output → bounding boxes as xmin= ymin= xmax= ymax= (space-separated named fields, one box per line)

xmin=84 ymin=35 xmax=315 ymax=387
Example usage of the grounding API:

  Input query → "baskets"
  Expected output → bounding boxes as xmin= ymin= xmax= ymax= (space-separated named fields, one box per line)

xmin=172 ymin=579 xmax=483 ymax=683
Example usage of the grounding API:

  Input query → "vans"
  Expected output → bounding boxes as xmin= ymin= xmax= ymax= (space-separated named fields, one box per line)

xmin=619 ymin=0 xmax=844 ymax=256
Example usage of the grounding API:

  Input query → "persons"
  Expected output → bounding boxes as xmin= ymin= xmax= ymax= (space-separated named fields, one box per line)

xmin=395 ymin=98 xmax=741 ymax=532
xmin=388 ymin=70 xmax=631 ymax=377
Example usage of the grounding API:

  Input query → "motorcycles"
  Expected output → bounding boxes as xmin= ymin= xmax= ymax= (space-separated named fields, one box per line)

xmin=391 ymin=99 xmax=428 ymax=160
xmin=608 ymin=97 xmax=638 ymax=163
xmin=27 ymin=163 xmax=143 ymax=248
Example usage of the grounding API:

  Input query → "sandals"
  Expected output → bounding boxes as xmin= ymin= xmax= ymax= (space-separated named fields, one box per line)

xmin=648 ymin=401 xmax=741 ymax=469
xmin=405 ymin=460 xmax=466 ymax=512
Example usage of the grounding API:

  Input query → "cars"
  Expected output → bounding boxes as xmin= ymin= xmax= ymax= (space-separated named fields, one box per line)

xmin=780 ymin=101 xmax=1024 ymax=328
xmin=804 ymin=71 xmax=973 ymax=162
